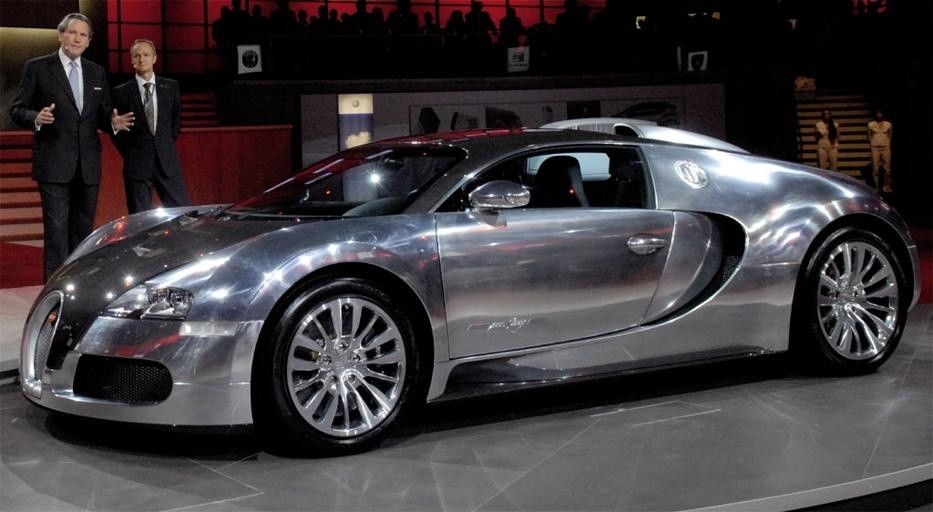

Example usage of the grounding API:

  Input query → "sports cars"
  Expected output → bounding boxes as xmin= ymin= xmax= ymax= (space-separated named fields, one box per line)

xmin=19 ymin=117 xmax=921 ymax=457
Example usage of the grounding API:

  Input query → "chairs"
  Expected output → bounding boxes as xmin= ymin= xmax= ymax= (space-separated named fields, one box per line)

xmin=598 ymin=158 xmax=646 ymax=208
xmin=533 ymin=155 xmax=587 ymax=209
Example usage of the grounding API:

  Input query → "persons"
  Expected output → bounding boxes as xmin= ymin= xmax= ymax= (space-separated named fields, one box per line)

xmin=867 ymin=107 xmax=893 ymax=193
xmin=210 ymin=2 xmax=592 ymax=81
xmin=815 ymin=108 xmax=840 ymax=171
xmin=111 ymin=39 xmax=188 ymax=216
xmin=8 ymin=13 xmax=136 ymax=285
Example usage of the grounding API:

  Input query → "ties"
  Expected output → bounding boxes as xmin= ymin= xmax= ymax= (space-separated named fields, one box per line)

xmin=143 ymin=83 xmax=155 ymax=135
xmin=69 ymin=61 xmax=81 ymax=116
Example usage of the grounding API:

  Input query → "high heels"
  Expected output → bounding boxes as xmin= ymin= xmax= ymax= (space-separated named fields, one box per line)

xmin=875 ymin=185 xmax=892 ymax=193
